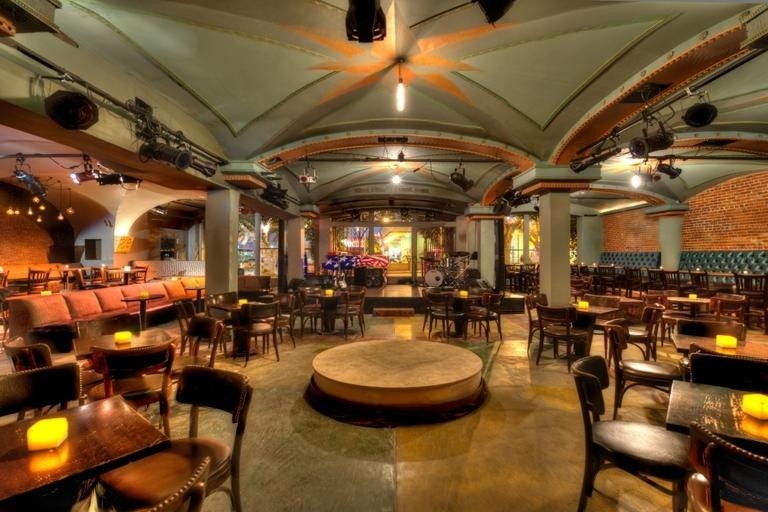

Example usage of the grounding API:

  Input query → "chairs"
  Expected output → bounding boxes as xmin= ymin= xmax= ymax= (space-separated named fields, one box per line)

xmin=524 ymin=293 xmax=570 ymax=360
xmin=98 ymin=366 xmax=253 ymax=510
xmin=677 ymin=319 xmax=747 ymax=343
xmin=693 ymin=422 xmax=766 ymax=511
xmin=167 ymin=315 xmax=223 ymax=381
xmin=3 ymin=363 xmax=80 ymax=423
xmin=535 ymin=303 xmax=589 ymax=373
xmin=505 ymin=246 xmax=767 ymax=329
xmin=572 ymin=357 xmax=692 ymax=509
xmin=418 ymin=245 xmax=504 ymax=343
xmin=689 ymin=352 xmax=766 ymax=394
xmin=604 ymin=319 xmax=679 ymax=422
xmin=608 ymin=309 xmax=662 ymax=367
xmin=3 ymin=337 xmax=103 ymax=421
xmin=118 ymin=458 xmax=210 ymax=511
xmin=175 ymin=247 xmax=391 ymax=365
xmin=75 ymin=313 xmax=142 ymax=337
xmin=1 ymin=244 xmax=175 ymax=338
xmin=87 ymin=343 xmax=175 ymax=437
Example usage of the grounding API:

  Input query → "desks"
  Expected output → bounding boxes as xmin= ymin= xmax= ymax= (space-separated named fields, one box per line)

xmin=73 ymin=329 xmax=176 ymax=399
xmin=670 ymin=333 xmax=766 ymax=359
xmin=664 ymin=378 xmax=766 ymax=464
xmin=560 ymin=304 xmax=620 ymax=363
xmin=2 ymin=394 xmax=172 ymax=511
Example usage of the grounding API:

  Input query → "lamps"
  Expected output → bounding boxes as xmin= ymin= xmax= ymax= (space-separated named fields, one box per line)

xmin=34 ymin=69 xmax=291 ymax=215
xmin=448 ymin=158 xmax=480 ymax=195
xmin=394 ymin=56 xmax=407 ymax=112
xmin=560 ymin=79 xmax=725 ymax=176
